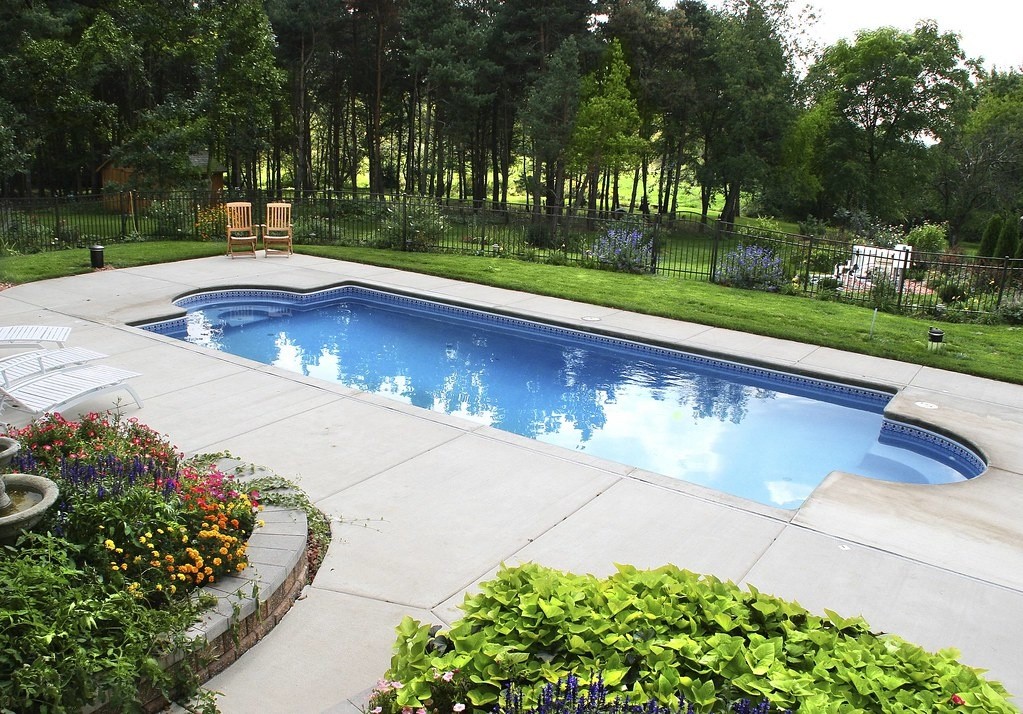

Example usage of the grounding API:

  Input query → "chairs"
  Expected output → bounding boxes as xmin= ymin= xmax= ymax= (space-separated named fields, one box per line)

xmin=261 ymin=203 xmax=293 ymax=258
xmin=0 ymin=325 xmax=71 ymax=350
xmin=0 ymin=364 xmax=143 ymax=427
xmin=0 ymin=347 xmax=111 ymax=401
xmin=226 ymin=202 xmax=257 ymax=260
